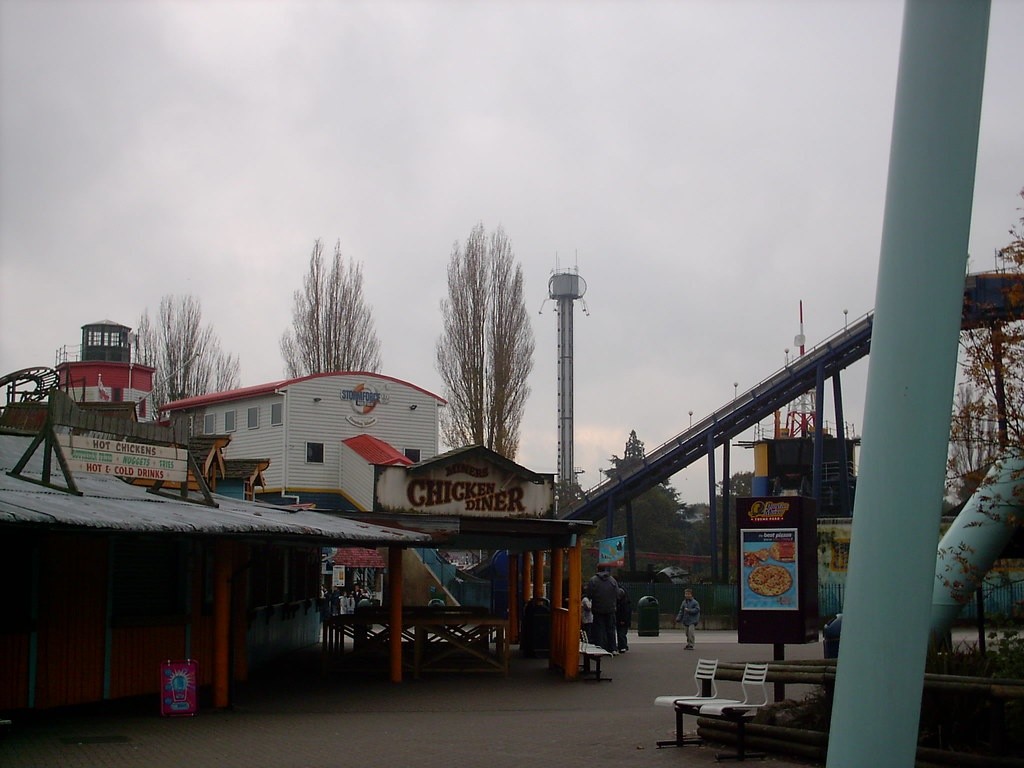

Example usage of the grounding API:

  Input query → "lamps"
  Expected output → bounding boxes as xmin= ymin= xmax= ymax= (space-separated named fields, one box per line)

xmin=410 ymin=404 xmax=417 ymax=410
xmin=314 ymin=397 xmax=321 ymax=402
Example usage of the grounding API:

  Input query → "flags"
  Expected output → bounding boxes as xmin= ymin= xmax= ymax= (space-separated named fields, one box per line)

xmin=99 ymin=382 xmax=109 ymax=401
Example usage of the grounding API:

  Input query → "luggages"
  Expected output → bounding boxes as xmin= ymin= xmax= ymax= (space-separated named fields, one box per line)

xmin=160 ymin=658 xmax=199 ymax=719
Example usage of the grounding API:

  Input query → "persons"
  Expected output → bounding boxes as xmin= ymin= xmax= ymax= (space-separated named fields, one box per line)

xmin=612 ymin=576 xmax=632 ymax=652
xmin=587 ymin=568 xmax=618 ymax=655
xmin=320 ymin=583 xmax=369 ymax=616
xmin=581 ymin=591 xmax=593 ymax=641
xmin=676 ymin=589 xmax=700 ymax=649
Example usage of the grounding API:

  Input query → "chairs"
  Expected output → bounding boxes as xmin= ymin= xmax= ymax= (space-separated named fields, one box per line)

xmin=654 ymin=657 xmax=770 ymax=761
xmin=579 ymin=630 xmax=613 ymax=682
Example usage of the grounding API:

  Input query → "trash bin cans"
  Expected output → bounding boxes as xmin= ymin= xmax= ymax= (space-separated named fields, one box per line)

xmin=822 ymin=614 xmax=842 ymax=695
xmin=519 ymin=596 xmax=551 ymax=660
xmin=637 ymin=596 xmax=659 ymax=637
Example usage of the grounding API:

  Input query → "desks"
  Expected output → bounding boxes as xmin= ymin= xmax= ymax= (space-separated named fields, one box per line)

xmin=322 ymin=606 xmax=511 ymax=679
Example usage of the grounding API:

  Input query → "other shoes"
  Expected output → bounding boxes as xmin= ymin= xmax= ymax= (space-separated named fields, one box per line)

xmin=684 ymin=646 xmax=694 ymax=650
xmin=620 ymin=649 xmax=625 ymax=653
xmin=612 ymin=651 xmax=620 ymax=655
